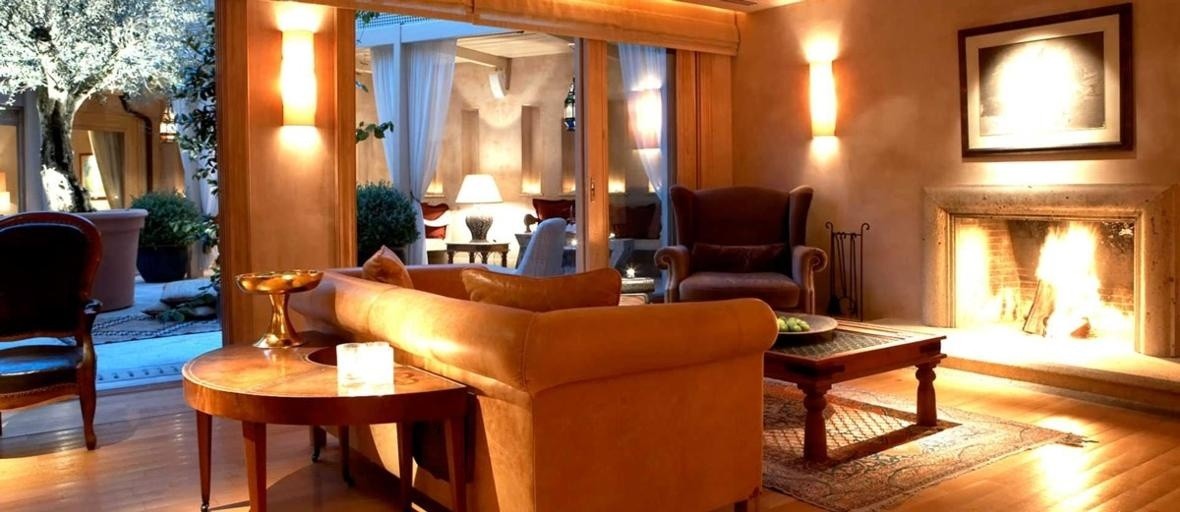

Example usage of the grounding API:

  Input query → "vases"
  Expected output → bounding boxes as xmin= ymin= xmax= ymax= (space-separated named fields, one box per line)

xmin=77 ymin=207 xmax=149 ymax=313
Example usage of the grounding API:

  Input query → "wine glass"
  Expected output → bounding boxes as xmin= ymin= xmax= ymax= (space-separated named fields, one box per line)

xmin=232 ymin=267 xmax=324 ymax=350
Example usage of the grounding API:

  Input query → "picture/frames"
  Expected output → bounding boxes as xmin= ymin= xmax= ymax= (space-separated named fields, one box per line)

xmin=78 ymin=152 xmax=108 ymax=200
xmin=958 ymin=0 xmax=1135 ymax=158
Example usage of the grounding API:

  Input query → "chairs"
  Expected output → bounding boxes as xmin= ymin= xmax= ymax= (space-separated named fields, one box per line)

xmin=530 ymin=195 xmax=663 ymax=237
xmin=484 ymin=216 xmax=567 ymax=277
xmin=0 ymin=206 xmax=110 ymax=450
xmin=656 ymin=181 xmax=826 ymax=310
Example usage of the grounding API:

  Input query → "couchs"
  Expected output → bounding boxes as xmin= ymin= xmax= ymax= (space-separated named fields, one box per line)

xmin=287 ymin=258 xmax=779 ymax=511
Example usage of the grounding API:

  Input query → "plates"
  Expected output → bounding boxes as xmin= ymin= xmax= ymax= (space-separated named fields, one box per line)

xmin=769 ymin=309 xmax=839 ymax=338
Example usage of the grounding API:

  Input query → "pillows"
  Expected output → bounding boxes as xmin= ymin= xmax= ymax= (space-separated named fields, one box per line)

xmin=356 ymin=240 xmax=414 ymax=291
xmin=459 ymin=259 xmax=623 ymax=311
xmin=688 ymin=236 xmax=787 ymax=273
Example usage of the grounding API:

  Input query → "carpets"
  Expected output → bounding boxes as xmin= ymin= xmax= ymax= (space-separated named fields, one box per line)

xmin=745 ymin=366 xmax=1089 ymax=512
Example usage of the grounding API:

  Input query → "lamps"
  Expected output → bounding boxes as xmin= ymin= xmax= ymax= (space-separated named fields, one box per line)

xmin=276 ymin=25 xmax=318 ymax=123
xmin=803 ymin=56 xmax=836 ymax=135
xmin=559 ymin=75 xmax=579 ymax=133
xmin=456 ymin=174 xmax=504 ymax=242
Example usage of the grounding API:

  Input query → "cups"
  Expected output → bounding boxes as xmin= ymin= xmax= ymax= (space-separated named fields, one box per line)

xmin=335 ymin=337 xmax=395 ymax=387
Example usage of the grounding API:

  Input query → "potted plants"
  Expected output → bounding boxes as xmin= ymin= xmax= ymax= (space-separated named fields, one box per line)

xmin=351 ymin=181 xmax=420 ymax=265
xmin=132 ymin=187 xmax=198 ymax=282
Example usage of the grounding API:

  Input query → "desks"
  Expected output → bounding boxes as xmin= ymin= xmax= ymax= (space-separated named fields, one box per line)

xmin=179 ymin=325 xmax=482 ymax=512
xmin=759 ymin=313 xmax=948 ymax=469
xmin=445 ymin=242 xmax=510 ymax=267
